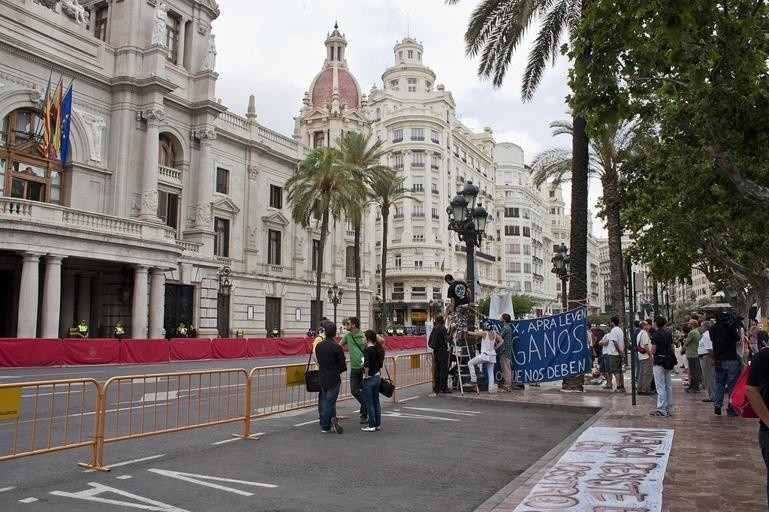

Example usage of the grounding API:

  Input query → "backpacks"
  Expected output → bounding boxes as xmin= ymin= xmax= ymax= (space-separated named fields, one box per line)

xmin=428 ymin=326 xmax=443 ymax=351
xmin=656 ymin=331 xmax=677 ymax=371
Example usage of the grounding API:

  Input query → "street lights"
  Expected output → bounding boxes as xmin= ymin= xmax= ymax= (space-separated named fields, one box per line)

xmin=446 ymin=179 xmax=488 ymax=390
xmin=551 ymin=242 xmax=570 ymax=313
xmin=543 ymin=293 xmax=562 ymax=318
xmin=624 ymin=294 xmax=654 ymax=321
xmin=327 ymin=284 xmax=343 ymax=336
xmin=429 ymin=298 xmax=442 ymax=323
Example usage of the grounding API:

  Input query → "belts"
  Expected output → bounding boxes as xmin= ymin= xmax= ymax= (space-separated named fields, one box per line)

xmin=699 ymin=353 xmax=710 ymax=357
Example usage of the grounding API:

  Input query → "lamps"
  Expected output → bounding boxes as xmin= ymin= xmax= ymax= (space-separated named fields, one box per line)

xmin=218 ymin=265 xmax=233 ymax=297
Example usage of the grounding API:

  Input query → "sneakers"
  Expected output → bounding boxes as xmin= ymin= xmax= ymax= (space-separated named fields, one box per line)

xmin=440 ymin=390 xmax=453 ymax=394
xmin=702 ymin=399 xmax=738 ymax=417
xmin=320 ymin=417 xmax=381 ymax=434
xmin=464 ymin=381 xmax=477 ymax=386
xmin=666 ymin=408 xmax=672 ymax=416
xmin=649 ymin=411 xmax=667 ymax=418
xmin=601 ymin=384 xmax=626 ymax=393
xmin=681 ymin=373 xmax=706 ymax=394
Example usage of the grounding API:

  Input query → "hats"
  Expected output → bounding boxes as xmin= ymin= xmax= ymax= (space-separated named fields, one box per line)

xmin=483 ymin=320 xmax=494 ymax=330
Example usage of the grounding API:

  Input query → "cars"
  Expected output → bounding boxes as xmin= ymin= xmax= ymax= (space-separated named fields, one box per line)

xmin=403 ymin=325 xmax=426 ymax=335
xmin=587 ymin=320 xmax=640 ymax=360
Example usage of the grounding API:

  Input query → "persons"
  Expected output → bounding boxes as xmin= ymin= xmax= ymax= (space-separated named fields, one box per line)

xmin=429 ymin=315 xmax=456 ymax=394
xmin=308 ymin=315 xmax=406 ymax=434
xmin=498 ymin=382 xmax=540 ymax=390
xmin=672 ymin=311 xmax=768 ymax=414
xmin=76 ymin=319 xmax=89 ymax=338
xmin=745 ymin=346 xmax=768 ymax=508
xmin=626 ymin=315 xmax=674 ymax=415
xmin=112 ymin=320 xmax=128 ymax=339
xmin=496 ymin=314 xmax=513 ymax=393
xmin=586 ymin=316 xmax=626 ymax=393
xmin=188 ymin=322 xmax=196 ymax=338
xmin=445 ymin=274 xmax=471 ymax=342
xmin=268 ymin=324 xmax=281 ymax=338
xmin=458 ymin=320 xmax=504 ymax=394
xmin=176 ymin=321 xmax=188 ymax=338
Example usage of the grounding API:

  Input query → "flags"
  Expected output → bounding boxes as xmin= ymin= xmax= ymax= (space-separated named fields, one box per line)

xmin=61 ymin=82 xmax=73 ymax=168
xmin=40 ymin=76 xmax=52 ymax=159
xmin=50 ymin=78 xmax=63 ymax=161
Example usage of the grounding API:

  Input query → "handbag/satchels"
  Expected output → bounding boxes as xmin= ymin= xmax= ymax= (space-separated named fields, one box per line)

xmin=637 ymin=334 xmax=646 ymax=354
xmin=379 ymin=375 xmax=395 ymax=397
xmin=304 ymin=370 xmax=324 ymax=392
xmin=728 ymin=346 xmax=766 ymax=418
